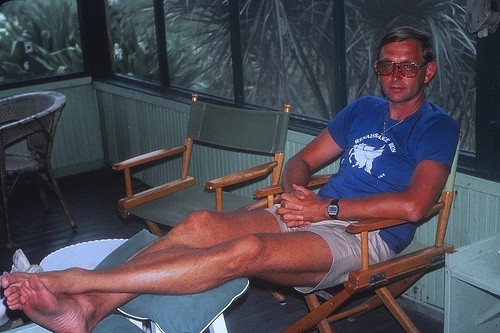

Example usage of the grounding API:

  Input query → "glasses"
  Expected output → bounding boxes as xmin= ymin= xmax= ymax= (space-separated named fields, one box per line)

xmin=375 ymin=59 xmax=428 ymax=78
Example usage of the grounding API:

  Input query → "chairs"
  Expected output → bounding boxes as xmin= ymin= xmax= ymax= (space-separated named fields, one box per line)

xmin=254 ymin=131 xmax=463 ymax=333
xmin=0 ymin=90 xmax=80 ymax=248
xmin=112 ymin=93 xmax=293 ymax=306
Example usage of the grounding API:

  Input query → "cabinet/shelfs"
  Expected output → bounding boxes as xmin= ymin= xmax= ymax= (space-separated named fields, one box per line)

xmin=443 ymin=234 xmax=500 ymax=333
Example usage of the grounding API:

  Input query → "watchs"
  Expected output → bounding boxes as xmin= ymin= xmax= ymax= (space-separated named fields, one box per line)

xmin=327 ymin=198 xmax=341 ymax=220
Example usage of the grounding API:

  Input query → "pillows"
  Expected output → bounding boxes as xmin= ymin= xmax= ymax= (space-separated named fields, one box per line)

xmin=92 ymin=229 xmax=250 ymax=333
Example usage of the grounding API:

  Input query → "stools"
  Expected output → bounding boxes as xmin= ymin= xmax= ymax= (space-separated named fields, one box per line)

xmin=125 ymin=310 xmax=228 ymax=333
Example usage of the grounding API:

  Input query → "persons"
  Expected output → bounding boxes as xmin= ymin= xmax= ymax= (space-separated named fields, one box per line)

xmin=0 ymin=28 xmax=461 ymax=333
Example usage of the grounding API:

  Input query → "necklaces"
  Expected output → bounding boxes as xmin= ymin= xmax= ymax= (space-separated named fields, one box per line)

xmin=377 ymin=109 xmax=406 ymax=140
